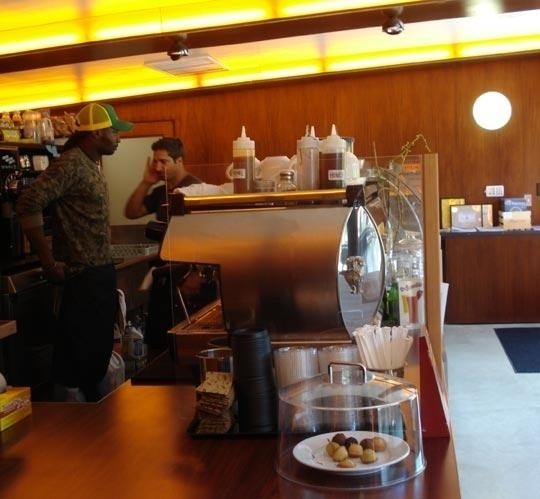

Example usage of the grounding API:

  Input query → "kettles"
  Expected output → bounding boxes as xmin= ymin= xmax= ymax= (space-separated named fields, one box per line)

xmin=32 ymin=118 xmax=55 ymax=144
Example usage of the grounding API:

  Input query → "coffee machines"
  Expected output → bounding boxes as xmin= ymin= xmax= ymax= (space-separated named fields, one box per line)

xmin=159 ymin=196 xmax=392 ymax=364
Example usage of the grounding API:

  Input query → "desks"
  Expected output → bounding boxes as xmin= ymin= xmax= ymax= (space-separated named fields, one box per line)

xmin=0 ymin=377 xmax=466 ymax=498
xmin=439 ymin=227 xmax=540 ymax=327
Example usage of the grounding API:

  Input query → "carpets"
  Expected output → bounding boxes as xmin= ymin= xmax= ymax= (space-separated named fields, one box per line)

xmin=493 ymin=326 xmax=539 ymax=372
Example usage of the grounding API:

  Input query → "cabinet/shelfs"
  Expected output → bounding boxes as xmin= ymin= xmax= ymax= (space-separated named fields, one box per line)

xmin=0 ymin=141 xmax=67 ymax=267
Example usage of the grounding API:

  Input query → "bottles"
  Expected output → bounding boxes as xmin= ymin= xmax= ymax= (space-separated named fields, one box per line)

xmin=231 ymin=328 xmax=278 ymax=433
xmin=388 ymin=276 xmax=400 ymax=327
xmin=297 ymin=122 xmax=346 ymax=205
xmin=255 ymin=180 xmax=275 ymax=207
xmin=276 ymin=171 xmax=296 ymax=206
xmin=232 ymin=126 xmax=256 ymax=205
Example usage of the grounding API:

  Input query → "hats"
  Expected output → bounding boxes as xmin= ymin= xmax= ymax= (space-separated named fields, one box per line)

xmin=73 ymin=102 xmax=133 ymax=131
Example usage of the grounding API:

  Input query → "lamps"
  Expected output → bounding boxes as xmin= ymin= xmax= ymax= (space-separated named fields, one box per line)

xmin=380 ymin=6 xmax=407 ymax=37
xmin=163 ymin=32 xmax=192 ymax=62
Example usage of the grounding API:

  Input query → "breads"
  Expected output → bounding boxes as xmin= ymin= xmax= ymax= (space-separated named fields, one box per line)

xmin=325 ymin=433 xmax=385 ymax=467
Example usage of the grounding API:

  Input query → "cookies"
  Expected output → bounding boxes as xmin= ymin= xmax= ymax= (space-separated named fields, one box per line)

xmin=195 ymin=370 xmax=235 ymax=435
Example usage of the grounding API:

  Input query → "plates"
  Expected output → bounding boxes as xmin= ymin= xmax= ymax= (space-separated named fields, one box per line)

xmin=292 ymin=430 xmax=411 ymax=477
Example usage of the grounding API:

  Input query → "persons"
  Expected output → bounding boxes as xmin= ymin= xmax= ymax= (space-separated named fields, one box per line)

xmin=15 ymin=102 xmax=134 ymax=399
xmin=124 ymin=136 xmax=204 ymax=357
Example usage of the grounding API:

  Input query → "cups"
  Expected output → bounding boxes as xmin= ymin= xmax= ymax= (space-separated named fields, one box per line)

xmin=207 ymin=336 xmax=229 ymax=349
xmin=196 ymin=348 xmax=233 ymax=383
xmin=402 ymin=294 xmax=419 ymax=324
xmin=225 ymin=156 xmax=296 ymax=184
